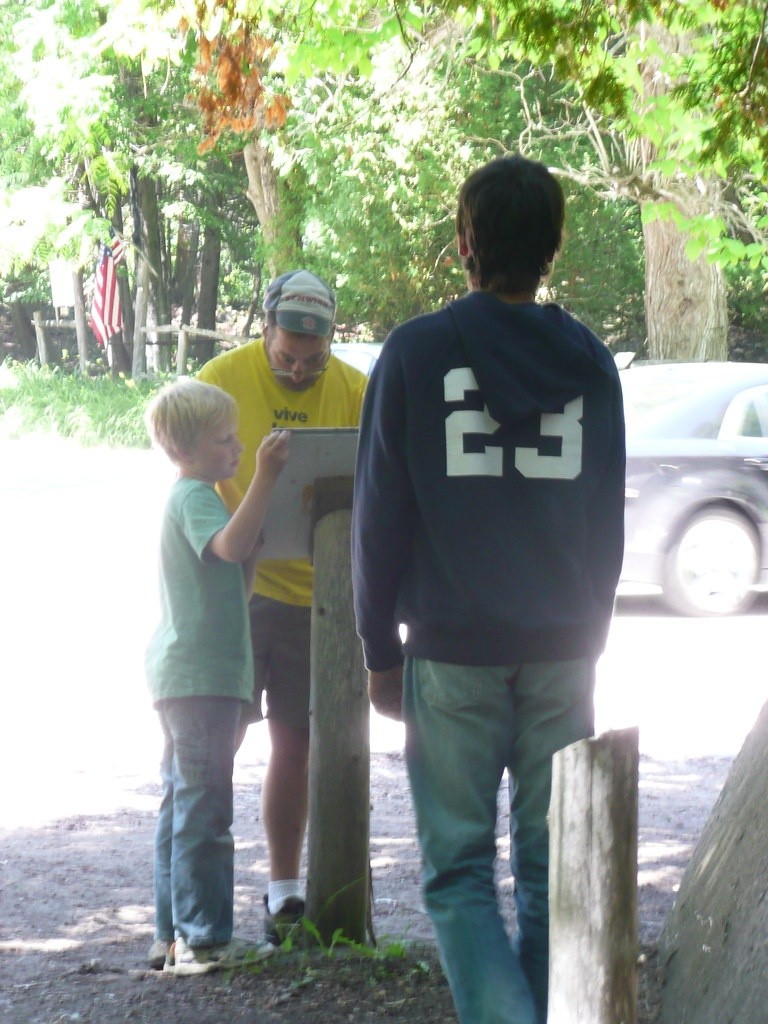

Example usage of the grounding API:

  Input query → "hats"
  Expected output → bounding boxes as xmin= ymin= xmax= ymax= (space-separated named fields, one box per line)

xmin=262 ymin=269 xmax=337 ymax=336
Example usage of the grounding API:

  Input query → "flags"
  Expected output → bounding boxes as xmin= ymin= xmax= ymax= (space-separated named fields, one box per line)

xmin=91 ymin=243 xmax=123 ymax=349
xmin=109 ymin=233 xmax=123 ymax=266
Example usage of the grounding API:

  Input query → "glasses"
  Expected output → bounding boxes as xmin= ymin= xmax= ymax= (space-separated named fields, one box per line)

xmin=269 ymin=369 xmax=328 ymax=381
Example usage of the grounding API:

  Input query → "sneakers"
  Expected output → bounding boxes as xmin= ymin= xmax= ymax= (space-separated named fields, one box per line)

xmin=161 ymin=952 xmax=174 ymax=972
xmin=173 ymin=936 xmax=275 ymax=977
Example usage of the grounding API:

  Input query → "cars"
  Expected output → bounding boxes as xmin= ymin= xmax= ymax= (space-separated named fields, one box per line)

xmin=329 ymin=338 xmax=392 ymax=382
xmin=608 ymin=360 xmax=768 ymax=620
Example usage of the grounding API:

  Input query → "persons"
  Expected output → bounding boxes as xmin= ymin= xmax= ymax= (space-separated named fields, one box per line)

xmin=351 ymin=152 xmax=627 ymax=1024
xmin=147 ymin=377 xmax=274 ymax=977
xmin=198 ymin=268 xmax=370 ymax=952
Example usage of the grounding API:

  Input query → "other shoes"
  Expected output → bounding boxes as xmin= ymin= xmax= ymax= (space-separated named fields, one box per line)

xmin=263 ymin=893 xmax=305 ymax=948
xmin=147 ymin=939 xmax=171 ymax=969
